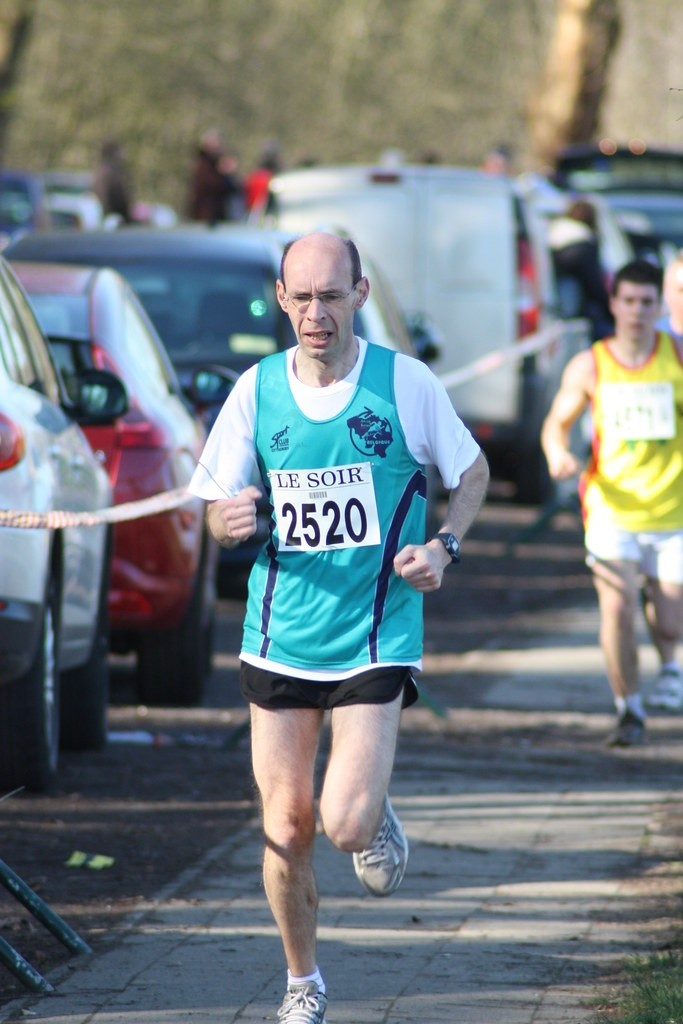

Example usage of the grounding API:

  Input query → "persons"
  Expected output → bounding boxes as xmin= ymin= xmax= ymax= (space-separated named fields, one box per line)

xmin=547 ymin=203 xmax=616 ymax=508
xmin=540 ymin=263 xmax=682 ymax=748
xmin=93 ymin=126 xmax=518 ymax=227
xmin=188 ymin=231 xmax=491 ymax=1023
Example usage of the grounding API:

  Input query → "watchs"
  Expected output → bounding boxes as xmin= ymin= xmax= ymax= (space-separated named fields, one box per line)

xmin=432 ymin=534 xmax=462 ymax=563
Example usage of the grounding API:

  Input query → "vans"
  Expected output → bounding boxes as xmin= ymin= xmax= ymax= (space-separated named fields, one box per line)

xmin=264 ymin=144 xmax=683 ymax=509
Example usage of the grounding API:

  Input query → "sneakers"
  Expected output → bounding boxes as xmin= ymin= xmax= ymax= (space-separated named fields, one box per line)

xmin=351 ymin=786 xmax=409 ymax=899
xmin=277 ymin=980 xmax=327 ymax=1024
xmin=606 ymin=709 xmax=644 ymax=747
xmin=646 ymin=662 xmax=683 ymax=711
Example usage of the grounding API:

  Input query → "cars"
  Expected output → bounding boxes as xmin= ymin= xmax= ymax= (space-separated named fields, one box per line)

xmin=0 ymin=166 xmax=442 ymax=794
